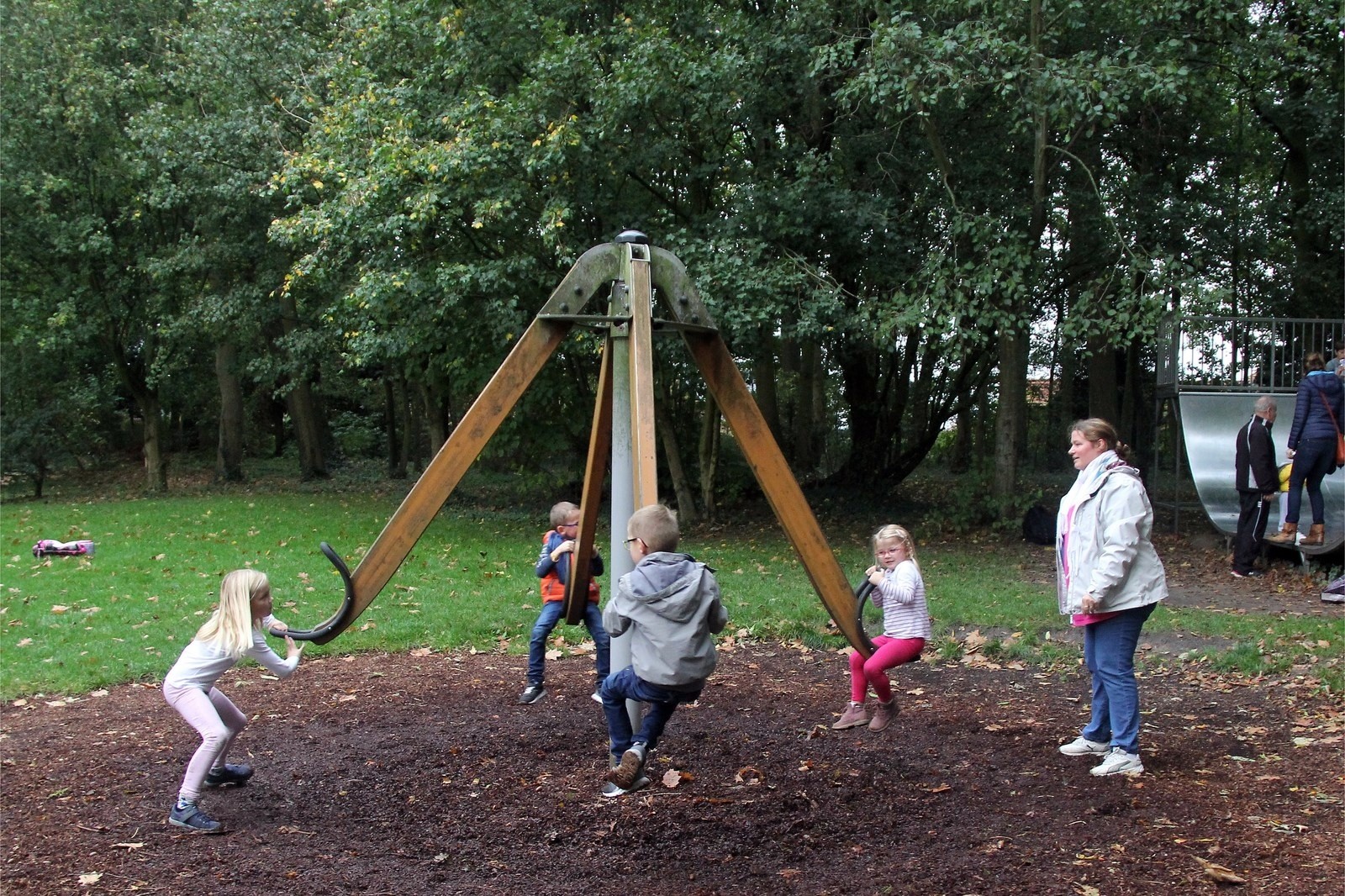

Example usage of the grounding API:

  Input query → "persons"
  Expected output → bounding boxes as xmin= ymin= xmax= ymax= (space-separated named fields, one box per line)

xmin=519 ymin=502 xmax=609 ymax=704
xmin=1230 ymin=396 xmax=1281 ymax=579
xmin=1268 ymin=341 xmax=1345 ymax=546
xmin=831 ymin=524 xmax=932 ymax=734
xmin=1054 ymin=418 xmax=1169 ymax=776
xmin=603 ymin=504 xmax=728 ymax=796
xmin=163 ymin=568 xmax=305 ymax=833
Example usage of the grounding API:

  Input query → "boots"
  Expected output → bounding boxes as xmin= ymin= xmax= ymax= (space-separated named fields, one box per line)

xmin=1267 ymin=522 xmax=1297 ymax=544
xmin=1299 ymin=524 xmax=1325 ymax=546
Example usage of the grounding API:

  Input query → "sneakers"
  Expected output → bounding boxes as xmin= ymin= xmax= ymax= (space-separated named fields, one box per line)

xmin=832 ymin=703 xmax=868 ymax=731
xmin=169 ymin=804 xmax=226 ymax=832
xmin=519 ymin=683 xmax=547 ymax=704
xmin=1090 ymin=747 xmax=1144 ymax=776
xmin=869 ymin=698 xmax=900 ymax=732
xmin=1231 ymin=568 xmax=1263 ymax=578
xmin=1059 ymin=728 xmax=1111 ymax=756
xmin=591 ymin=687 xmax=603 ymax=704
xmin=204 ymin=763 xmax=253 ymax=786
xmin=602 ymin=775 xmax=650 ymax=798
xmin=607 ymin=745 xmax=647 ymax=790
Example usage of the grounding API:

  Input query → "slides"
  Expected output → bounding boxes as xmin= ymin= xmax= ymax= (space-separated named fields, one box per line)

xmin=1179 ymin=393 xmax=1344 ymax=555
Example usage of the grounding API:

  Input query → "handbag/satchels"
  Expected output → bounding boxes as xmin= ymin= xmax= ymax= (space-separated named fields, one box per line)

xmin=1337 ymin=434 xmax=1345 ymax=469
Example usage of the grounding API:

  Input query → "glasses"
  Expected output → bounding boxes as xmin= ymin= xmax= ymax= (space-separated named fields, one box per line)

xmin=623 ymin=537 xmax=648 ymax=550
xmin=558 ymin=522 xmax=578 ymax=527
xmin=875 ymin=546 xmax=906 ymax=558
